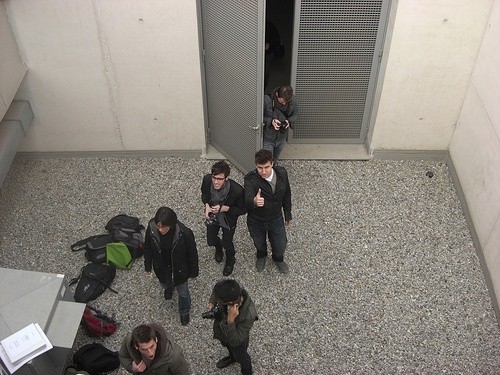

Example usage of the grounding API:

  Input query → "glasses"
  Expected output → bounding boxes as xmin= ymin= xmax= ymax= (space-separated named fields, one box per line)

xmin=154 ymin=225 xmax=167 ymax=229
xmin=211 ymin=175 xmax=225 ymax=182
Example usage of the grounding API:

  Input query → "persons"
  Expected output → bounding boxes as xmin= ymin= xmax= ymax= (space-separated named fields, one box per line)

xmin=200 ymin=160 xmax=247 ymax=277
xmin=244 ymin=148 xmax=293 ymax=275
xmin=265 ymin=19 xmax=287 ymax=60
xmin=118 ymin=321 xmax=193 ymax=375
xmin=143 ymin=206 xmax=199 ymax=326
xmin=208 ymin=277 xmax=259 ymax=375
xmin=263 ymin=84 xmax=299 ymax=166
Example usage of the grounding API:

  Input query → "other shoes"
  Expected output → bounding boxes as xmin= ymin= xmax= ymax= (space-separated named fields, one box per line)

xmin=181 ymin=314 xmax=190 ymax=325
xmin=164 ymin=288 xmax=173 ymax=300
xmin=223 ymin=261 xmax=235 ymax=276
xmin=216 ymin=356 xmax=233 ymax=368
xmin=276 ymin=261 xmax=289 ymax=274
xmin=255 ymin=257 xmax=265 ymax=272
xmin=215 ymin=246 xmax=224 ymax=262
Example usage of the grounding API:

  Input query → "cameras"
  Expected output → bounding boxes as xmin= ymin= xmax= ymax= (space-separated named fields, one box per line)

xmin=205 ymin=213 xmax=218 ymax=228
xmin=201 ymin=304 xmax=224 ymax=321
xmin=277 ymin=121 xmax=287 ymax=134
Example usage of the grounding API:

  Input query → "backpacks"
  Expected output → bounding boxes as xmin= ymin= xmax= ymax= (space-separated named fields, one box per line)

xmin=80 ymin=306 xmax=117 ymax=340
xmin=69 ymin=262 xmax=118 ymax=303
xmin=71 ymin=214 xmax=145 ymax=270
xmin=63 ymin=341 xmax=120 ymax=374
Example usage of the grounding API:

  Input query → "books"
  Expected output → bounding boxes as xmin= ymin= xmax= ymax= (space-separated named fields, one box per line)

xmin=0 ymin=322 xmax=54 ymax=375
